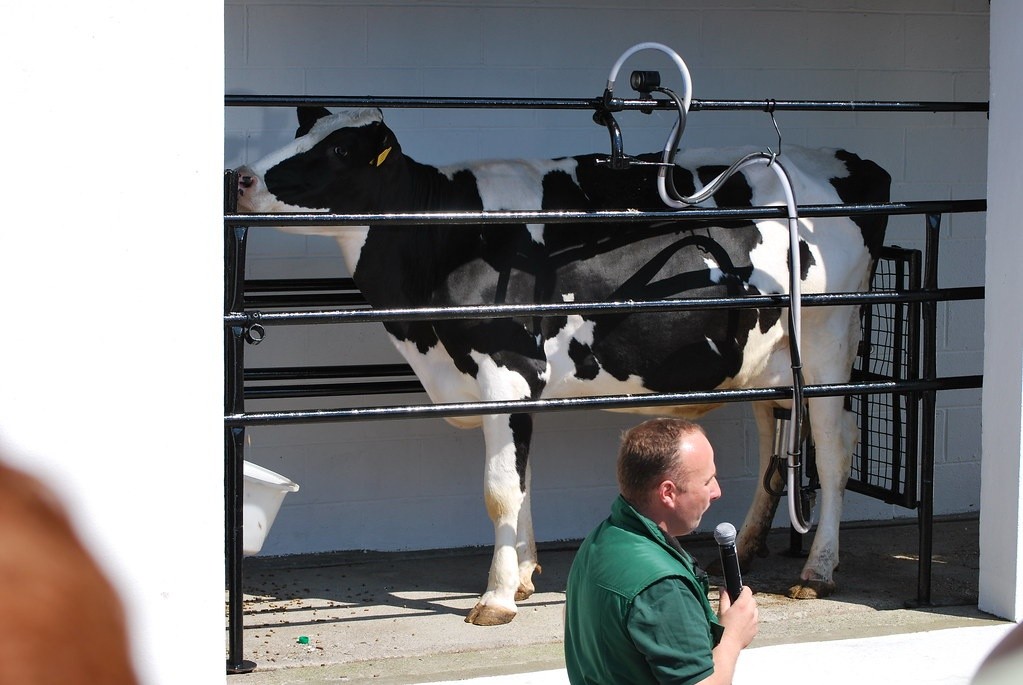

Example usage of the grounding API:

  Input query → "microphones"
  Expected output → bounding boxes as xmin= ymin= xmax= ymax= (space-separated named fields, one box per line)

xmin=713 ymin=522 xmax=744 ymax=605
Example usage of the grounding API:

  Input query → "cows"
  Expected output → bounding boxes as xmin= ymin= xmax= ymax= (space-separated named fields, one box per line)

xmin=234 ymin=105 xmax=895 ymax=626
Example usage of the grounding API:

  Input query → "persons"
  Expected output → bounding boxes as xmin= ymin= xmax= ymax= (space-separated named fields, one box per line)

xmin=0 ymin=466 xmax=141 ymax=685
xmin=563 ymin=418 xmax=759 ymax=685
